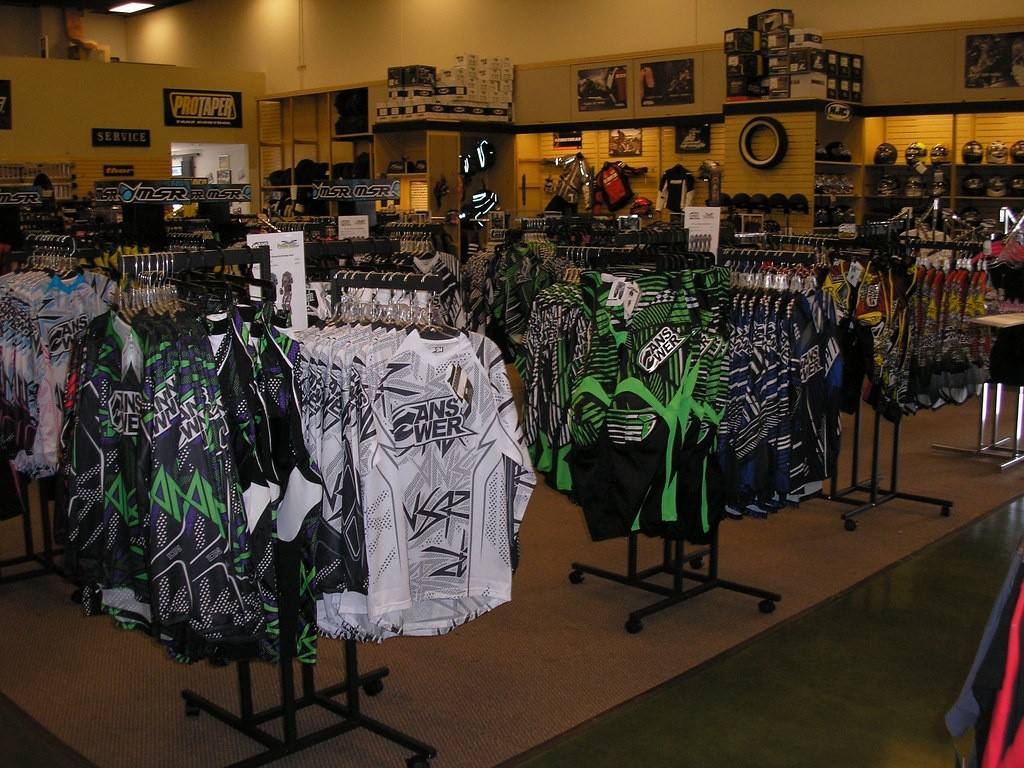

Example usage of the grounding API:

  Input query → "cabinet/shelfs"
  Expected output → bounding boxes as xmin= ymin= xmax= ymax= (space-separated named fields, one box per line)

xmin=954 ymin=163 xmax=1024 ymax=200
xmin=862 ymin=163 xmax=951 ymax=199
xmin=814 ymin=159 xmax=863 ymax=230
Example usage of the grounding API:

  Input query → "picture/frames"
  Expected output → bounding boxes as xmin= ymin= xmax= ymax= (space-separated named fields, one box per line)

xmin=218 ymin=154 xmax=230 ymax=170
xmin=217 ymin=169 xmax=231 ymax=184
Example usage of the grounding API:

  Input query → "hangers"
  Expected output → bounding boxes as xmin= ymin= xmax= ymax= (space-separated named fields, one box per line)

xmin=476 ymin=216 xmax=1024 ymax=297
xmin=111 ymin=218 xmax=277 ymax=325
xmin=325 ymin=271 xmax=460 ymax=342
xmin=378 ymin=237 xmax=402 ymax=272
xmin=309 ymin=238 xmax=332 ymax=280
xmin=277 ymin=216 xmax=337 ymax=237
xmin=342 ymin=238 xmax=377 ymax=272
xmin=19 ymin=212 xmax=103 ymax=280
xmin=359 ymin=236 xmax=382 ymax=271
xmin=384 ymin=222 xmax=437 ymax=268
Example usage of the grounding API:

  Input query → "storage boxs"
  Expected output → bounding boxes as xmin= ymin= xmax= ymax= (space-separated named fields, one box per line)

xmin=375 ymin=50 xmax=515 ymax=123
xmin=723 ymin=8 xmax=864 ymax=103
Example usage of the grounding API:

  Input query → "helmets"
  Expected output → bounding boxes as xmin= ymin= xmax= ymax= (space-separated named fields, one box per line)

xmin=962 ymin=141 xmax=984 ymax=165
xmin=750 ymin=194 xmax=769 ymax=214
xmin=987 ymin=176 xmax=1007 ymax=198
xmin=1010 ymin=140 xmax=1024 ymax=164
xmin=986 ymin=140 xmax=1009 ymax=165
xmin=732 ymin=193 xmax=752 ymax=211
xmin=768 ymin=193 xmax=788 ymax=214
xmin=630 ymin=197 xmax=653 ymax=215
xmin=961 ymin=174 xmax=985 ymax=196
xmin=877 ymin=175 xmax=899 ymax=198
xmin=930 ymin=175 xmax=949 ymax=196
xmin=717 ymin=193 xmax=731 ymax=209
xmin=904 ymin=141 xmax=928 ymax=166
xmin=905 ymin=176 xmax=926 ymax=197
xmin=1009 ymin=174 xmax=1024 ymax=198
xmin=874 ymin=143 xmax=898 ymax=165
xmin=813 ymin=139 xmax=857 ymax=226
xmin=930 ymin=142 xmax=952 ymax=165
xmin=787 ymin=194 xmax=809 ymax=214
